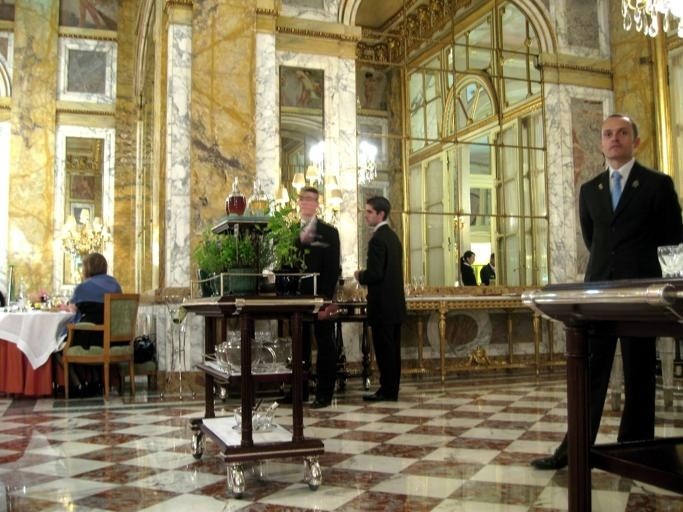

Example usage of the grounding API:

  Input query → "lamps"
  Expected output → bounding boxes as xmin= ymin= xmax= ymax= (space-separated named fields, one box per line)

xmin=622 ymin=0 xmax=683 ymax=41
xmin=308 ymin=140 xmax=340 ymax=179
xmin=358 ymin=140 xmax=378 ymax=184
xmin=292 ymin=165 xmax=343 ymax=223
xmin=62 ymin=209 xmax=111 ymax=261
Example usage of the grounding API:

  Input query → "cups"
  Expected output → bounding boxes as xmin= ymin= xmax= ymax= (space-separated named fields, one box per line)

xmin=214 ymin=342 xmax=227 ymax=365
xmin=657 ymin=246 xmax=683 ymax=279
xmin=404 ymin=276 xmax=424 ymax=297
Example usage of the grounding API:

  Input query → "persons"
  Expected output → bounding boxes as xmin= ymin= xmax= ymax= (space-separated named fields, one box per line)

xmin=272 ymin=187 xmax=339 ymax=408
xmin=57 ymin=252 xmax=130 ymax=398
xmin=459 ymin=250 xmax=477 ymax=286
xmin=480 ymin=253 xmax=494 ymax=286
xmin=353 ymin=196 xmax=408 ymax=403
xmin=530 ymin=114 xmax=682 ymax=469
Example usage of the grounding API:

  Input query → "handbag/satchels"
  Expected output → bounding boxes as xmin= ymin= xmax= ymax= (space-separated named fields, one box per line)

xmin=134 ymin=338 xmax=154 ymax=363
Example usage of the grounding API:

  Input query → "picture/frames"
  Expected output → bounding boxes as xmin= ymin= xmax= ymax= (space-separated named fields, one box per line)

xmin=66 ymin=170 xmax=98 ymax=203
xmin=280 ymin=64 xmax=323 ymax=113
xmin=357 ymin=65 xmax=390 ymax=116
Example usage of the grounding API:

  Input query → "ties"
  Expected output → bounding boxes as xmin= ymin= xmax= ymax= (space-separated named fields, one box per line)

xmin=610 ymin=173 xmax=623 ymax=207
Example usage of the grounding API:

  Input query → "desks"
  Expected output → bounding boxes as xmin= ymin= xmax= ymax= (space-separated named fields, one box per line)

xmin=217 ymin=301 xmax=374 ymax=402
xmin=521 ymin=277 xmax=683 ymax=512
xmin=1 ymin=303 xmax=73 ymax=398
xmin=404 ymin=294 xmax=555 ymax=384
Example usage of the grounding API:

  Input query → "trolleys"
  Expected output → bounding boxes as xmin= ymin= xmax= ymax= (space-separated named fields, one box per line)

xmin=326 ymin=298 xmax=376 ymax=396
xmin=181 ymin=176 xmax=332 ymax=500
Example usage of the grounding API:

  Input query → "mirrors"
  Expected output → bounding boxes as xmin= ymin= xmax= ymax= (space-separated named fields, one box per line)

xmin=280 ymin=63 xmax=323 ymax=208
xmin=60 ymin=136 xmax=104 ymax=287
xmin=357 ymin=0 xmax=549 ymax=288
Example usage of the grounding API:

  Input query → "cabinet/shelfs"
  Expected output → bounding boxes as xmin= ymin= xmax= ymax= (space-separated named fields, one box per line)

xmin=177 ymin=292 xmax=325 ymax=498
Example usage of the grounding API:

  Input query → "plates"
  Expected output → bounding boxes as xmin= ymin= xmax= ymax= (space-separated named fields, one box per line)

xmin=232 ymin=425 xmax=277 ymax=433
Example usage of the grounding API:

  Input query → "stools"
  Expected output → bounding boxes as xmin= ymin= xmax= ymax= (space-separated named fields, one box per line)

xmin=117 ymin=360 xmax=156 ymax=397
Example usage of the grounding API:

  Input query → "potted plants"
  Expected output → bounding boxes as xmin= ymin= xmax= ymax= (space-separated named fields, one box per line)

xmin=195 ymin=200 xmax=311 ymax=297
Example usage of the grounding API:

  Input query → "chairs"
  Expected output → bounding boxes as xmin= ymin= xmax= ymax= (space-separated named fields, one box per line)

xmin=64 ymin=293 xmax=140 ymax=400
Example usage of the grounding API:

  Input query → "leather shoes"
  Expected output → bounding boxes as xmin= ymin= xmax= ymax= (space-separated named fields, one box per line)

xmin=363 ymin=391 xmax=399 ymax=402
xmin=55 ymin=381 xmax=90 ymax=399
xmin=277 ymin=396 xmax=309 ymax=405
xmin=311 ymin=399 xmax=332 ymax=409
xmin=532 ymin=451 xmax=569 ymax=470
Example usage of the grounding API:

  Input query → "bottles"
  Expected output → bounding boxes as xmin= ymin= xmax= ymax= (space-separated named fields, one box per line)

xmin=489 ymin=274 xmax=495 ymax=288
xmin=225 ymin=176 xmax=269 ymax=216
xmin=8 ymin=264 xmax=16 ymax=312
